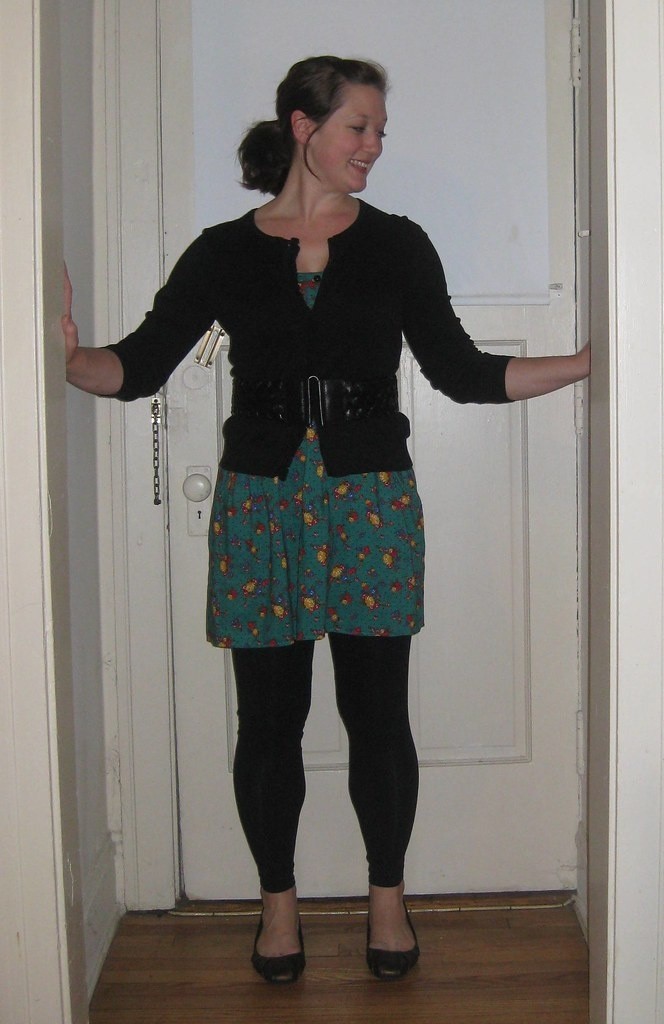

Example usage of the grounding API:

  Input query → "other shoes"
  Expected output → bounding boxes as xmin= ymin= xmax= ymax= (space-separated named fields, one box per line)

xmin=367 ymin=897 xmax=420 ymax=982
xmin=251 ymin=907 xmax=306 ymax=985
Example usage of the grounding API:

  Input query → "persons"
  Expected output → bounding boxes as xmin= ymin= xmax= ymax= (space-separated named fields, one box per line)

xmin=61 ymin=56 xmax=592 ymax=983
